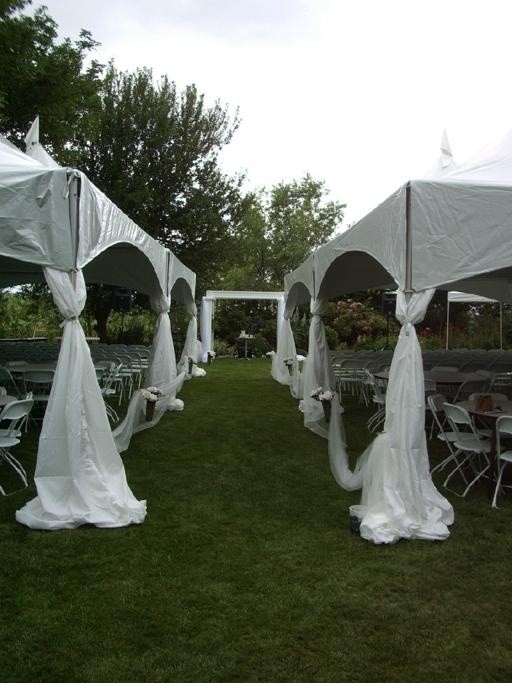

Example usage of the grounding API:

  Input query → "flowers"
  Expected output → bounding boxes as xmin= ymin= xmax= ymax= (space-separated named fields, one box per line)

xmin=309 ymin=386 xmax=336 ymax=401
xmin=140 ymin=387 xmax=165 ymax=403
xmin=283 ymin=359 xmax=293 ymax=366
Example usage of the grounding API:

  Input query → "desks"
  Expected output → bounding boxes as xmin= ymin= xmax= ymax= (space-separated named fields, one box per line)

xmin=239 ymin=338 xmax=255 ymax=361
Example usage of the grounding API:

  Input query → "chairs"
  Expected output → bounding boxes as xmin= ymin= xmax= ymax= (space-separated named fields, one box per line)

xmin=330 ymin=348 xmax=512 ymax=509
xmin=0 ymin=342 xmax=152 ymax=496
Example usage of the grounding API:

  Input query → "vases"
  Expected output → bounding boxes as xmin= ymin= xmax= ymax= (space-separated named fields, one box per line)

xmin=146 ymin=400 xmax=156 ymax=421
xmin=322 ymin=399 xmax=331 ymax=423
xmin=288 ymin=365 xmax=292 ymax=376
